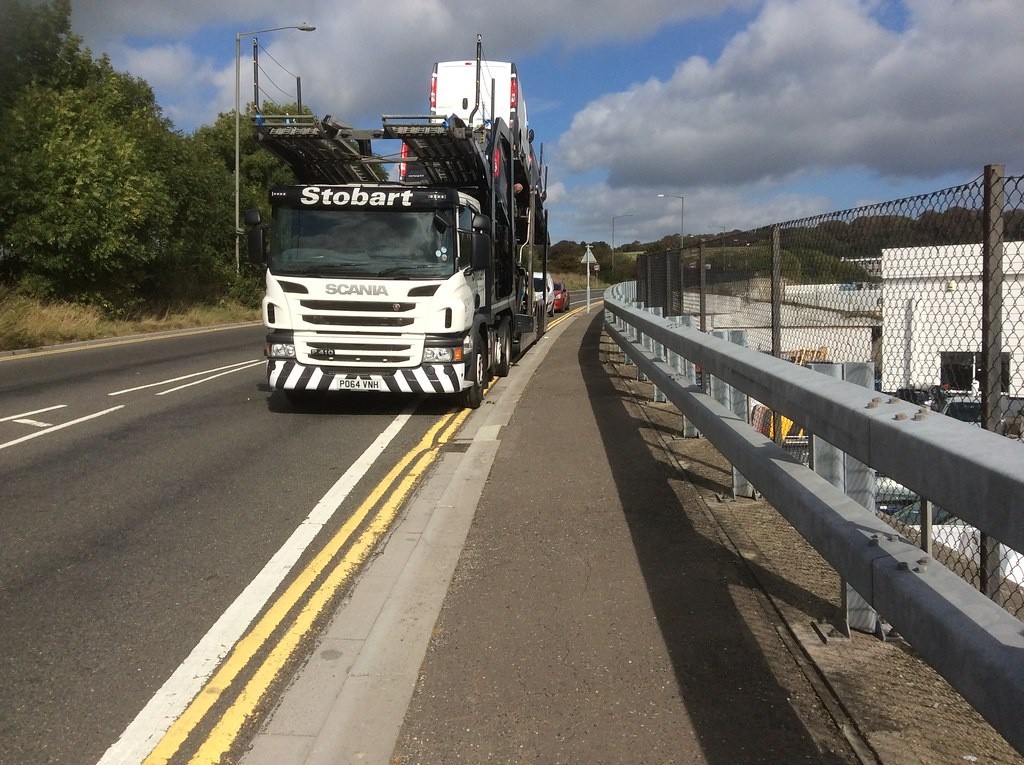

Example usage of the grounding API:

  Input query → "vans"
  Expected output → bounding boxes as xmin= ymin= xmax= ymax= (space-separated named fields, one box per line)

xmin=399 ymin=126 xmax=523 ymax=254
xmin=429 ymin=59 xmax=547 ymax=218
xmin=526 ymin=272 xmax=555 ymax=317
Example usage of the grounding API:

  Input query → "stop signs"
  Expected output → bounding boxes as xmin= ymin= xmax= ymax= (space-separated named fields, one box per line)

xmin=594 ymin=264 xmax=600 ymax=271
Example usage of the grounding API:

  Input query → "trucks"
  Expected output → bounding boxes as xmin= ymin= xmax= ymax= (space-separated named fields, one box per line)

xmin=243 ymin=109 xmax=549 ymax=410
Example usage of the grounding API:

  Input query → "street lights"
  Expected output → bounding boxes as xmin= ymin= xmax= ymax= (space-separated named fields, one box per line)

xmin=234 ymin=22 xmax=317 ymax=277
xmin=612 ymin=214 xmax=634 ymax=271
xmin=658 ymin=193 xmax=684 ymax=247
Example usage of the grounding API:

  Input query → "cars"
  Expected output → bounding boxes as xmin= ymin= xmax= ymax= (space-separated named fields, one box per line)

xmin=890 ymin=499 xmax=1024 ymax=623
xmin=1001 ymin=396 xmax=1024 ymax=441
xmin=939 ymin=397 xmax=1006 ymax=432
xmin=553 ymin=283 xmax=570 ymax=312
